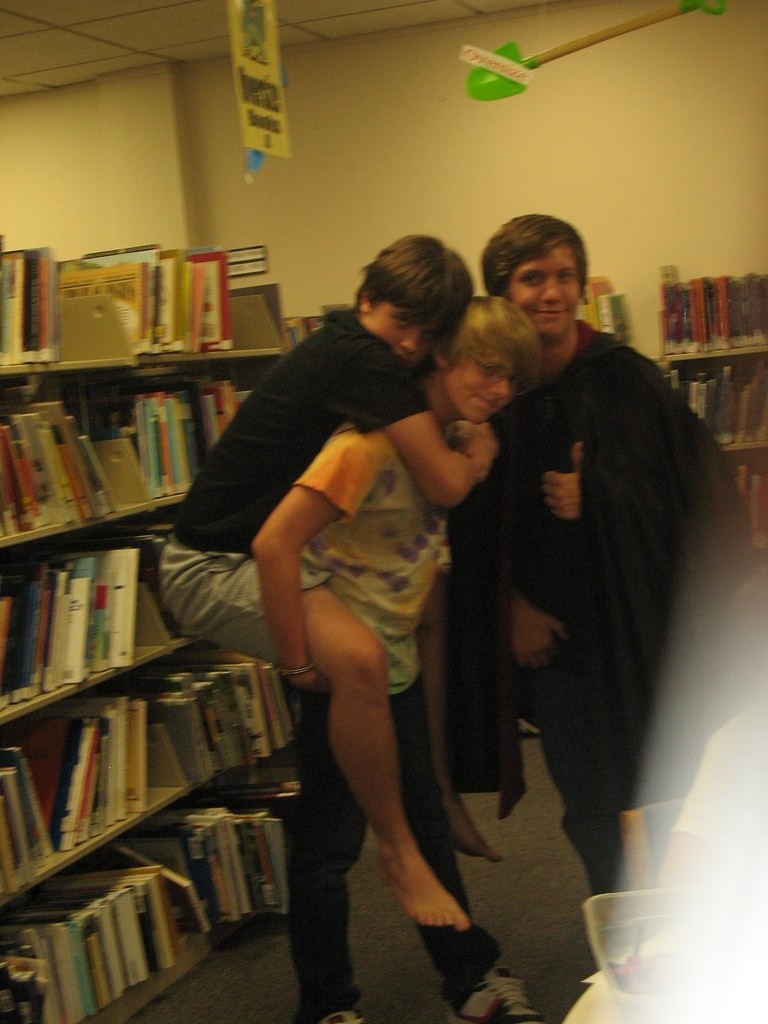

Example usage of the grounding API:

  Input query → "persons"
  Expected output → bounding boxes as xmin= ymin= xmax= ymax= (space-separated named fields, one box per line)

xmin=250 ymin=298 xmax=545 ymax=1024
xmin=158 ymin=234 xmax=508 ymax=931
xmin=480 ymin=213 xmax=755 ymax=895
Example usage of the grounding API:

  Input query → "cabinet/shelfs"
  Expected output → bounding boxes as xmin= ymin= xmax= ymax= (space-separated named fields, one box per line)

xmin=646 ymin=344 xmax=768 ymax=559
xmin=0 ymin=345 xmax=293 ymax=1024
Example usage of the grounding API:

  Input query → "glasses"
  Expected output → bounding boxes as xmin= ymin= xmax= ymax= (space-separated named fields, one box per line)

xmin=468 ymin=352 xmax=531 ymax=395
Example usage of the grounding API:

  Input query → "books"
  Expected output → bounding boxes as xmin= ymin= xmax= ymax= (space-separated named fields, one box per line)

xmin=0 ymin=660 xmax=302 ymax=1024
xmin=656 ymin=272 xmax=768 ymax=446
xmin=0 ymin=232 xmax=237 ymax=533
xmin=0 ymin=547 xmax=140 ymax=711
xmin=578 ymin=276 xmax=632 ymax=345
xmin=279 ymin=315 xmax=318 ymax=354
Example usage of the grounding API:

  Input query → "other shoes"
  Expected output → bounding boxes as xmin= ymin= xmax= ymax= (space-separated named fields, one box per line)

xmin=318 ymin=1007 xmax=367 ymax=1024
xmin=446 ymin=964 xmax=545 ymax=1024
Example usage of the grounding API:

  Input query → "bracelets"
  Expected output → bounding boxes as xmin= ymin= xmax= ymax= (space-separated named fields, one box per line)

xmin=288 ymin=662 xmax=316 ymax=676
